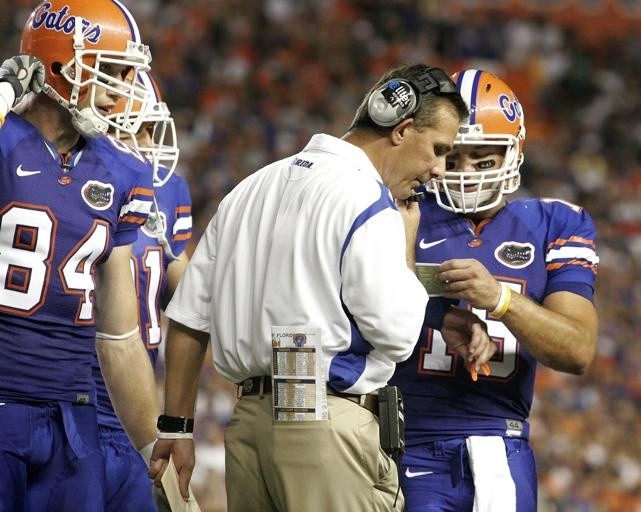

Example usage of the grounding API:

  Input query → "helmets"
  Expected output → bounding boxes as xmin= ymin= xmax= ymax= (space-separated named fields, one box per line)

xmin=20 ymin=0 xmax=151 ymax=139
xmin=106 ymin=63 xmax=180 ymax=187
xmin=421 ymin=68 xmax=526 ymax=216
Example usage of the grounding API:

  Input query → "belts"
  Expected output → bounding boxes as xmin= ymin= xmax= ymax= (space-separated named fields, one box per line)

xmin=237 ymin=374 xmax=380 ymax=418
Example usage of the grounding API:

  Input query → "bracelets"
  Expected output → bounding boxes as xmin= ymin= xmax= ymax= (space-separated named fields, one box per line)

xmin=490 ymin=281 xmax=508 ymax=317
xmin=155 ymin=414 xmax=195 ymax=434
xmin=155 ymin=431 xmax=195 ymax=441
xmin=423 ymin=296 xmax=451 ymax=332
xmin=494 ymin=284 xmax=511 ymax=321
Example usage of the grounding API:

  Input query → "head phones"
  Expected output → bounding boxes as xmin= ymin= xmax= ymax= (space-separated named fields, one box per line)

xmin=366 ymin=65 xmax=454 ymax=131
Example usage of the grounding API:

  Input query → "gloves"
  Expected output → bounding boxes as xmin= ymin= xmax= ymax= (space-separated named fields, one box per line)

xmin=0 ymin=55 xmax=45 ymax=128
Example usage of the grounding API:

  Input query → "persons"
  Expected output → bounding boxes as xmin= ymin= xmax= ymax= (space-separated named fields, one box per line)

xmin=92 ymin=65 xmax=193 ymax=512
xmin=0 ymin=0 xmax=175 ymax=511
xmin=379 ymin=70 xmax=599 ymax=512
xmin=148 ymin=62 xmax=469 ymax=512
